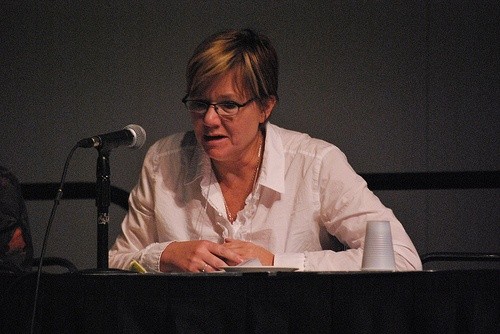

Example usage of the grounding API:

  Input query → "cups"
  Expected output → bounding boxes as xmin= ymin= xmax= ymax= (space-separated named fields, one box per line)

xmin=360 ymin=221 xmax=395 ymax=271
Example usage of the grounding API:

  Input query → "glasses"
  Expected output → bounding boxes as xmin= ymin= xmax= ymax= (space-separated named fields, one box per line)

xmin=182 ymin=94 xmax=257 ymax=117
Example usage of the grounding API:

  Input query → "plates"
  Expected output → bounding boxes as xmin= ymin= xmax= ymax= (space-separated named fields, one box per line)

xmin=219 ymin=267 xmax=298 ymax=272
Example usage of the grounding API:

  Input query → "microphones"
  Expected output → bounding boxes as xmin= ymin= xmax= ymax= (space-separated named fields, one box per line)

xmin=78 ymin=123 xmax=146 ymax=151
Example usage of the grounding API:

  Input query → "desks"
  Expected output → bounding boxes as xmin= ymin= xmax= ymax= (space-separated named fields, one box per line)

xmin=1 ymin=269 xmax=500 ymax=334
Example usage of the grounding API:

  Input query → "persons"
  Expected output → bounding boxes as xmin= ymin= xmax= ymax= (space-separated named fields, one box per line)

xmin=0 ymin=164 xmax=34 ymax=276
xmin=108 ymin=26 xmax=423 ymax=274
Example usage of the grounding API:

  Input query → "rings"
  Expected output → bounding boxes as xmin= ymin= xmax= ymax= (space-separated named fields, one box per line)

xmin=201 ymin=263 xmax=209 ymax=272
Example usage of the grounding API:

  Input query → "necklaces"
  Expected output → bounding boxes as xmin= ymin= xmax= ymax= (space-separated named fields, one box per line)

xmin=211 ymin=137 xmax=262 ymax=222
